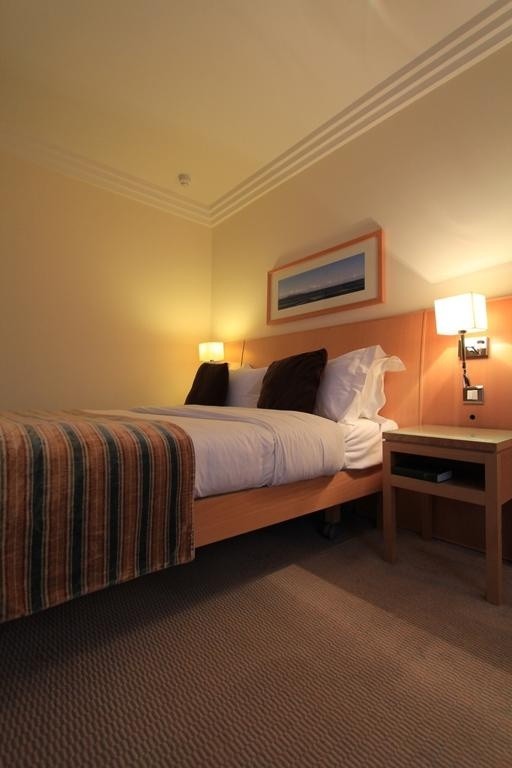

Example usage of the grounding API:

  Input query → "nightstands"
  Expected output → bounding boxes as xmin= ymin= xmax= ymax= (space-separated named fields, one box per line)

xmin=382 ymin=423 xmax=512 ymax=605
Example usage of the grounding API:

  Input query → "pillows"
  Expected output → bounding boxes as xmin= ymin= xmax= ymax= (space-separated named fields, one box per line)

xmin=184 ymin=346 xmax=385 ymax=426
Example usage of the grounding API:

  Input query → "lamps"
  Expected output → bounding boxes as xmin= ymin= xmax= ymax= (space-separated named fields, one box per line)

xmin=433 ymin=292 xmax=487 ymax=389
xmin=198 ymin=341 xmax=224 ymax=364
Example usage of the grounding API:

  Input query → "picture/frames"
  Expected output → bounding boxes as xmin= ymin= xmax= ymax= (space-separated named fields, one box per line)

xmin=265 ymin=227 xmax=386 ymax=326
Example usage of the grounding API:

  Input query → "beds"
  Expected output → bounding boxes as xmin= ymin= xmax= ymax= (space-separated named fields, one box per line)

xmin=0 ymin=406 xmax=399 ymax=626
